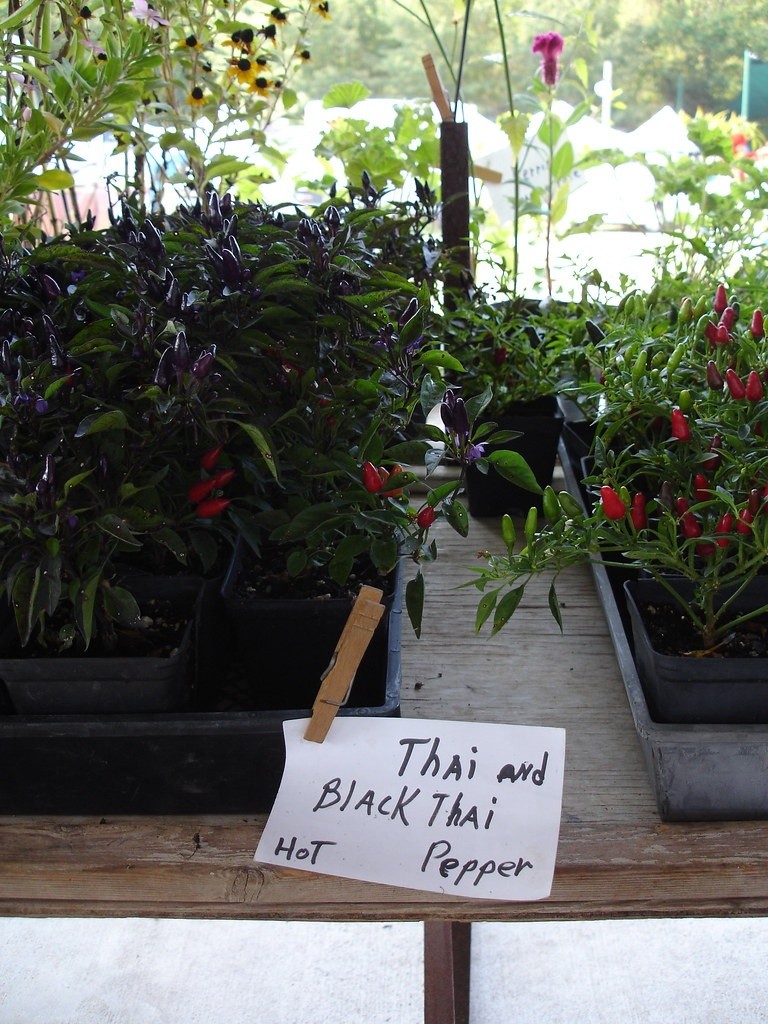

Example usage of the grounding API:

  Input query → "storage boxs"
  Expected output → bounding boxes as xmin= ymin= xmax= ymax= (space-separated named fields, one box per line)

xmin=558 ymin=435 xmax=768 ymax=822
xmin=0 ymin=519 xmax=405 ymax=818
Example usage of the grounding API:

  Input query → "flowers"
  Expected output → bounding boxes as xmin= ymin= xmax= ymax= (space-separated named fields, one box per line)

xmin=0 ymin=0 xmax=767 ymax=303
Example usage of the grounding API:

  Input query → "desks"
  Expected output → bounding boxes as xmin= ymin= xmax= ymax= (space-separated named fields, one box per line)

xmin=0 ymin=488 xmax=768 ymax=1024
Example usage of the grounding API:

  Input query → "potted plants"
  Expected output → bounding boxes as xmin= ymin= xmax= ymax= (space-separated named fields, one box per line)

xmin=0 ymin=172 xmax=767 ymax=724
